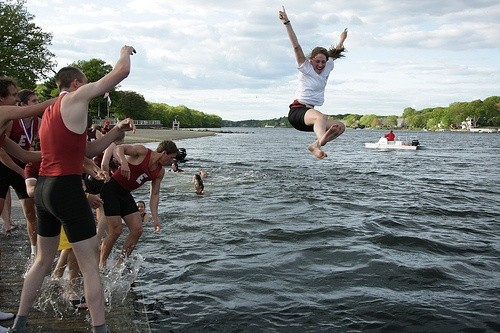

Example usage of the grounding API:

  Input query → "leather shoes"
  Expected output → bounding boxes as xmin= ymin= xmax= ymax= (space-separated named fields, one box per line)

xmin=0 ymin=325 xmax=8 ymax=333
xmin=0 ymin=311 xmax=14 ymax=321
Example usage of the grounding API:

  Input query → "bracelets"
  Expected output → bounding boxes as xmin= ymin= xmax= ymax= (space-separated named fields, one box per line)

xmin=283 ymin=20 xmax=290 ymax=25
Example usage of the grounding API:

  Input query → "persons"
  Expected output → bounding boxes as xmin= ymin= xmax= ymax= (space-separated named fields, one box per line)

xmin=168 ymin=162 xmax=184 ymax=172
xmin=0 ymin=44 xmax=178 ymax=333
xmin=192 ymin=170 xmax=206 ymax=195
xmin=279 ymin=4 xmax=349 ymax=161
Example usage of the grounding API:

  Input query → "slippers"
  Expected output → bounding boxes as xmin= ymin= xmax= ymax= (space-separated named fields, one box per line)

xmin=69 ymin=296 xmax=88 ymax=310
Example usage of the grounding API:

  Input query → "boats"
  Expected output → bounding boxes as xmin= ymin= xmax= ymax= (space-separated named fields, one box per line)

xmin=363 ymin=136 xmax=427 ymax=150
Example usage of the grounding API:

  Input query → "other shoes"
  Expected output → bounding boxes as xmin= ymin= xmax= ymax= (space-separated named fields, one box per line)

xmin=23 ymin=261 xmax=35 ymax=279
